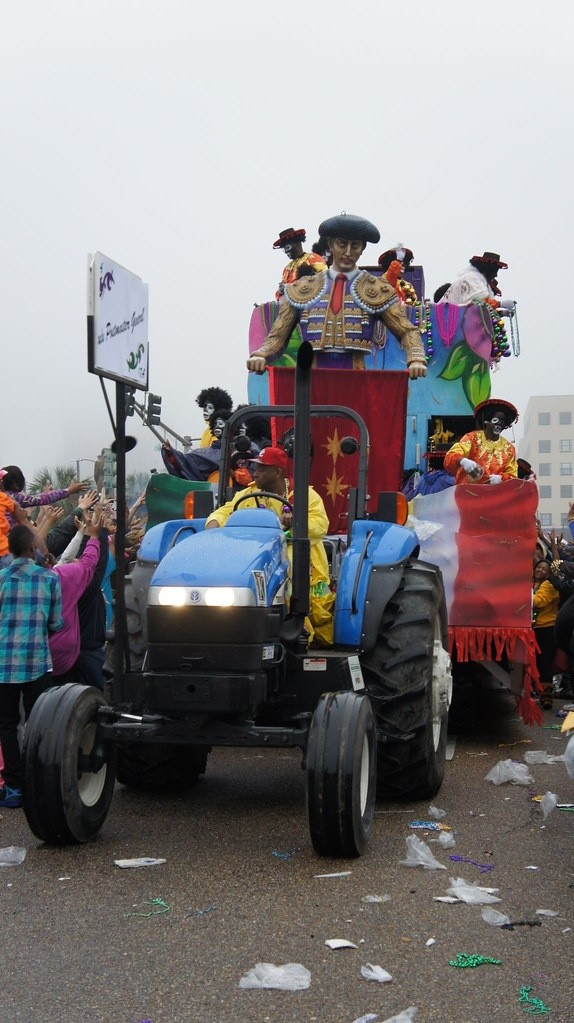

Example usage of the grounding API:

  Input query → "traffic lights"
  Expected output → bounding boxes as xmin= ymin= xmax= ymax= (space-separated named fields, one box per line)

xmin=124 ymin=384 xmax=137 ymax=415
xmin=146 ymin=394 xmax=162 ymax=425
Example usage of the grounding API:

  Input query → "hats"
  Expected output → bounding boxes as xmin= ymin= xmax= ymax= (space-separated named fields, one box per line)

xmin=273 ymin=228 xmax=306 ymax=249
xmin=474 ymin=399 xmax=519 ymax=431
xmin=422 ymin=443 xmax=454 ymax=458
xmin=378 ymin=248 xmax=414 ymax=265
xmin=433 ymin=283 xmax=451 ymax=303
xmin=0 ymin=466 xmax=25 ymax=493
xmin=247 ymin=447 xmax=289 ymax=470
xmin=516 ymin=458 xmax=538 ymax=481
xmin=490 ymin=278 xmax=501 ymax=294
xmin=469 ymin=252 xmax=509 ymax=270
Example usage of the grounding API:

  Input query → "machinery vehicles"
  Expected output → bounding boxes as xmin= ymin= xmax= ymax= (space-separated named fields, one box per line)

xmin=18 ymin=341 xmax=454 ymax=860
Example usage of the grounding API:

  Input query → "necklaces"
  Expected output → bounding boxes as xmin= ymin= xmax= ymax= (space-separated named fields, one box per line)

xmin=475 ymin=298 xmax=511 ymax=362
xmin=413 ymin=302 xmax=433 ymax=362
xmin=508 ymin=308 xmax=520 ymax=356
xmin=372 ymin=322 xmax=387 ymax=351
xmin=264 ymin=478 xmax=289 ymax=522
xmin=533 ymin=579 xmax=545 ymax=592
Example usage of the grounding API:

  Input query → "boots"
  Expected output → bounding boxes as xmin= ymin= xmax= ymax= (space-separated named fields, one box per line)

xmin=540 ymin=682 xmax=553 ymax=708
xmin=554 ymin=673 xmax=574 ymax=699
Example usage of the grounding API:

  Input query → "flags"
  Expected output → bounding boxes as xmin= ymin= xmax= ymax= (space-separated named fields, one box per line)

xmin=267 ymin=364 xmax=409 ymax=535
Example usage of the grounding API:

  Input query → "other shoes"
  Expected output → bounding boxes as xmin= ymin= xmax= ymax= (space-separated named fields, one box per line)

xmin=297 ymin=633 xmax=308 ymax=645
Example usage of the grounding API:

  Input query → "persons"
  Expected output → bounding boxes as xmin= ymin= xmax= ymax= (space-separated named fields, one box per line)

xmin=247 ymin=211 xmax=427 ymax=379
xmin=438 ymin=252 xmax=517 ymax=317
xmin=161 ymin=386 xmax=272 ymax=492
xmin=205 ymin=448 xmax=337 ymax=648
xmin=375 ymin=241 xmax=417 ymax=306
xmin=0 ymin=466 xmax=148 ymax=809
xmin=531 ymin=503 xmax=574 ymax=712
xmin=402 ymin=398 xmax=535 ymax=502
xmin=274 ymin=226 xmax=328 ymax=302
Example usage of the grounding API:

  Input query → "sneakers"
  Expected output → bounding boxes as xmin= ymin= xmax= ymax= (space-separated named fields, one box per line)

xmin=0 ymin=784 xmax=23 ymax=808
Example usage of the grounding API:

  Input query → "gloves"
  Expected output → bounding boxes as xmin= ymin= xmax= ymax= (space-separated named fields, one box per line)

xmin=395 ymin=243 xmax=406 ymax=262
